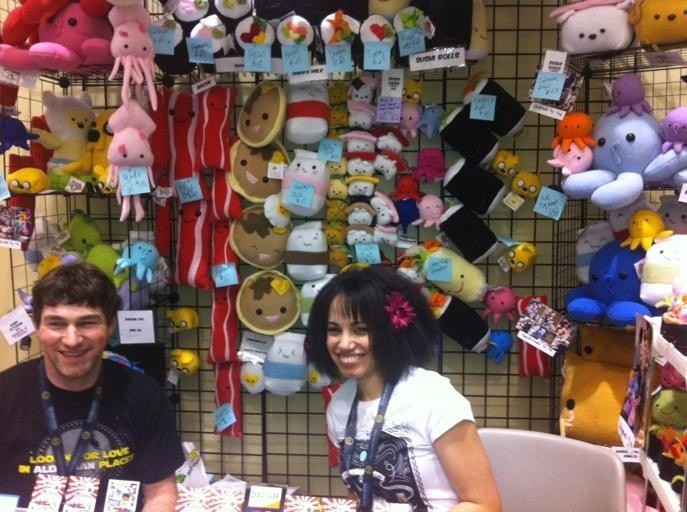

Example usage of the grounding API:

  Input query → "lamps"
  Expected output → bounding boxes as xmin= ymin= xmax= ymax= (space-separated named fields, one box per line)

xmin=462 ymin=429 xmax=624 ymax=512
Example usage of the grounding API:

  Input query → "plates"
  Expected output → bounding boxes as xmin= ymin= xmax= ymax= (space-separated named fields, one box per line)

xmin=518 ymin=31 xmax=686 ymax=440
xmin=624 ymin=313 xmax=685 ymax=512
xmin=0 ymin=0 xmax=180 ymax=431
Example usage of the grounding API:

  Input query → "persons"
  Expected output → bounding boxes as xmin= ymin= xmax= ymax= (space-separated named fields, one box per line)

xmin=0 ymin=263 xmax=186 ymax=512
xmin=303 ymin=266 xmax=501 ymax=511
